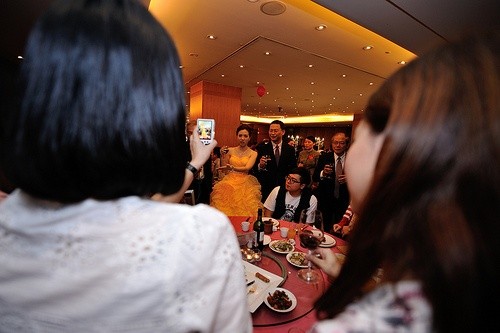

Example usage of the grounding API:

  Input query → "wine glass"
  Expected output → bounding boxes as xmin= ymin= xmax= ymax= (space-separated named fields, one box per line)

xmin=326 ymin=163 xmax=335 ymax=178
xmin=297 ymin=208 xmax=324 ymax=285
xmin=264 ymin=155 xmax=271 ymax=171
xmin=223 ymin=145 xmax=228 ymax=160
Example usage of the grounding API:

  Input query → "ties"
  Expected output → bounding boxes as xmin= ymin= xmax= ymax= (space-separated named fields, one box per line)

xmin=274 ymin=145 xmax=280 ymax=167
xmin=334 ymin=157 xmax=342 ymax=199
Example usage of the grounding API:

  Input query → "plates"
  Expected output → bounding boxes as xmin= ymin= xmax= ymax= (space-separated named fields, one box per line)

xmin=262 ymin=217 xmax=280 ymax=227
xmin=263 ymin=234 xmax=271 ymax=245
xmin=319 ymin=235 xmax=336 ymax=247
xmin=269 ymin=239 xmax=294 ymax=253
xmin=286 ymin=252 xmax=309 ymax=268
xmin=264 ymin=287 xmax=297 ymax=313
xmin=336 ymin=253 xmax=346 ymax=263
xmin=242 ymin=259 xmax=284 ymax=314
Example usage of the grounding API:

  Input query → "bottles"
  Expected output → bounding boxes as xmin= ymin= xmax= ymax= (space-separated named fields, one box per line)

xmin=253 ymin=208 xmax=263 ymax=252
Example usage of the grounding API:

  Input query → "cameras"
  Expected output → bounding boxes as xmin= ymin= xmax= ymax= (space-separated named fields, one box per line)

xmin=197 ymin=118 xmax=215 ymax=145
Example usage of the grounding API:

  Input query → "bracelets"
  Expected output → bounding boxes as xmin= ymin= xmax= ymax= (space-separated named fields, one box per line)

xmin=185 ymin=163 xmax=198 ymax=175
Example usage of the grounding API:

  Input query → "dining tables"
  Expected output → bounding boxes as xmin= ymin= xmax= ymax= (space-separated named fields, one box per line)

xmin=228 ymin=216 xmax=347 ymax=333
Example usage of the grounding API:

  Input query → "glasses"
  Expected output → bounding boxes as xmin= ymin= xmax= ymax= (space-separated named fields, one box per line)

xmin=284 ymin=175 xmax=301 ymax=184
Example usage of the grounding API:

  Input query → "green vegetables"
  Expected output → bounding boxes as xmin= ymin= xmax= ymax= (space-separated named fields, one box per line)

xmin=272 ymin=242 xmax=291 ymax=249
xmin=291 ymin=252 xmax=305 ymax=262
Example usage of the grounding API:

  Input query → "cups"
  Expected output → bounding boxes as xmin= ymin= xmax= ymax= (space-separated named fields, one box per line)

xmin=263 ymin=221 xmax=273 ymax=234
xmin=241 ymin=221 xmax=250 ymax=231
xmin=240 ymin=248 xmax=261 ymax=262
xmin=280 ymin=227 xmax=289 ymax=238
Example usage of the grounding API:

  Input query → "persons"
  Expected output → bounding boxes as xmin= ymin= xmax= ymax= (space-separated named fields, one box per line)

xmin=186 ymin=120 xmax=213 ymax=205
xmin=0 ymin=0 xmax=255 ymax=332
xmin=312 ymin=132 xmax=350 ymax=232
xmin=264 ymin=167 xmax=318 ymax=227
xmin=333 ymin=205 xmax=354 ymax=237
xmin=306 ymin=40 xmax=500 ymax=333
xmin=248 ymin=120 xmax=297 ymax=204
xmin=211 ymin=147 xmax=221 ymax=177
xmin=210 ymin=124 xmax=262 ymax=216
xmin=297 ymin=136 xmax=320 ymax=183
